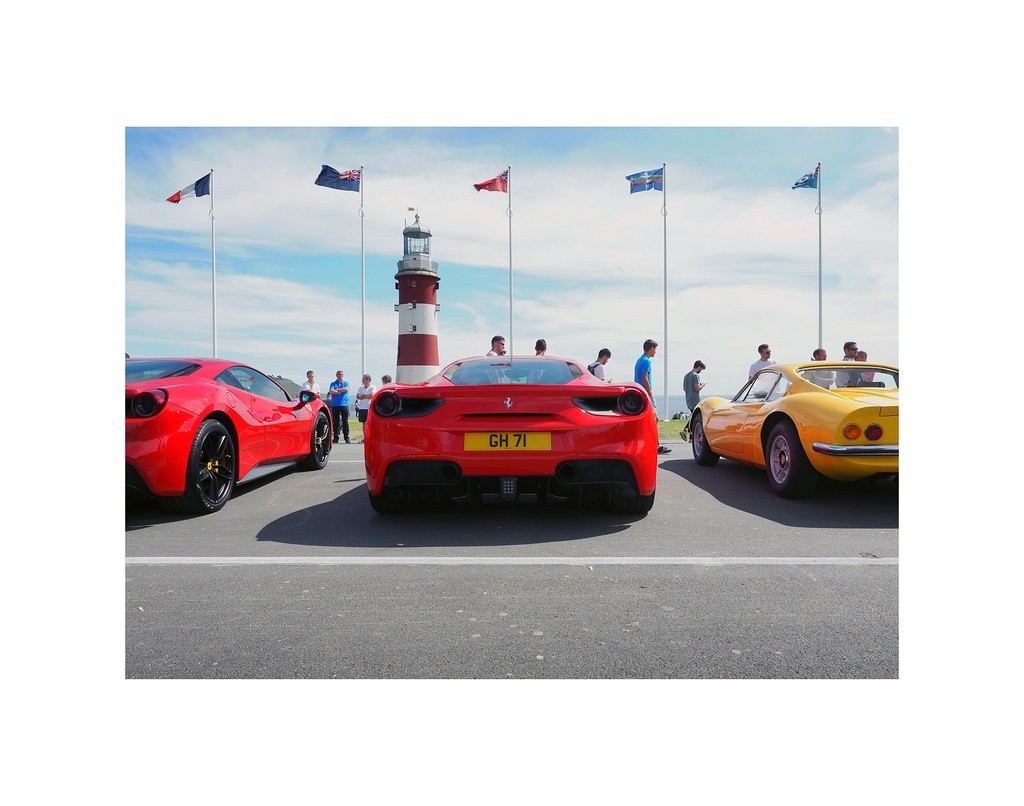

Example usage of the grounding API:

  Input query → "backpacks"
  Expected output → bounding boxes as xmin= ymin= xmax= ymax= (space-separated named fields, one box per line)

xmin=588 ymin=364 xmax=601 ymax=376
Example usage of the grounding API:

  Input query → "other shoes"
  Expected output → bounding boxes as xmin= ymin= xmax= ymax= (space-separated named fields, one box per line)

xmin=332 ymin=439 xmax=338 ymax=443
xmin=345 ymin=438 xmax=351 ymax=443
xmin=658 ymin=446 xmax=672 ymax=455
xmin=679 ymin=431 xmax=688 ymax=442
xmin=358 ymin=439 xmax=364 ymax=444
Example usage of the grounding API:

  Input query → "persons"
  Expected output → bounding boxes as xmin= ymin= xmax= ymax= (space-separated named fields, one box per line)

xmin=588 ymin=347 xmax=613 ymax=382
xmin=485 ymin=336 xmax=508 ymax=383
xmin=672 ymin=411 xmax=690 ymax=419
xmin=302 ymin=370 xmax=321 ymax=400
xmin=835 ymin=343 xmax=858 ymax=387
xmin=634 ymin=339 xmax=673 ymax=455
xmin=854 ymin=351 xmax=875 ymax=381
xmin=355 ymin=400 xmax=360 ymax=417
xmin=329 ymin=369 xmax=351 ymax=443
xmin=680 ymin=360 xmax=707 ymax=443
xmin=527 ymin=339 xmax=547 ymax=384
xmin=381 ymin=374 xmax=392 ymax=385
xmin=423 ymin=244 xmax=428 ymax=252
xmin=356 ymin=374 xmax=376 ymax=444
xmin=322 ymin=395 xmax=334 ymax=435
xmin=804 ymin=349 xmax=835 ymax=389
xmin=750 ymin=345 xmax=781 ymax=393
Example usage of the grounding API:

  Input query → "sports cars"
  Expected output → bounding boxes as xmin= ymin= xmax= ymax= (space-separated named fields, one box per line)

xmin=688 ymin=361 xmax=899 ymax=500
xmin=126 ymin=358 xmax=333 ymax=517
xmin=364 ymin=356 xmax=658 ymax=517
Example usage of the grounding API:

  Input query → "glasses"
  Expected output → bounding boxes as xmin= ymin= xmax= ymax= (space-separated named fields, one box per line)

xmin=847 ymin=348 xmax=858 ymax=351
xmin=762 ymin=350 xmax=771 ymax=354
xmin=500 ymin=343 xmax=505 ymax=346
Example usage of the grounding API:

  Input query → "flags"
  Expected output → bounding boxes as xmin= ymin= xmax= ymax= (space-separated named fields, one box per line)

xmin=166 ymin=171 xmax=210 ymax=205
xmin=314 ymin=164 xmax=360 ymax=193
xmin=791 ymin=166 xmax=818 ymax=190
xmin=473 ymin=169 xmax=508 ymax=192
xmin=625 ymin=167 xmax=663 ymax=194
